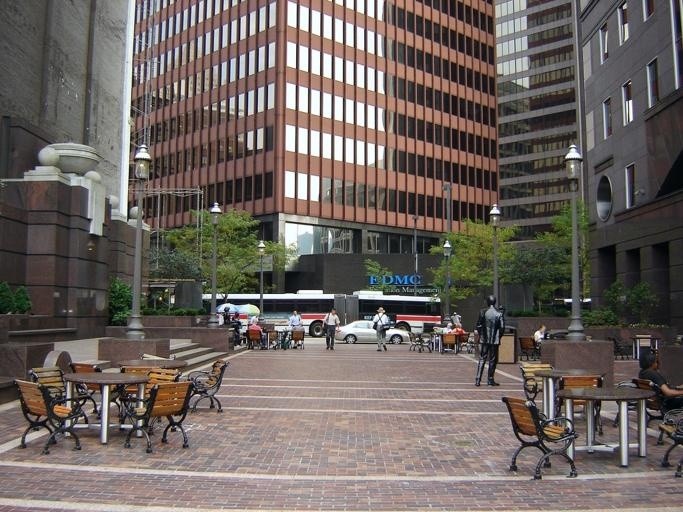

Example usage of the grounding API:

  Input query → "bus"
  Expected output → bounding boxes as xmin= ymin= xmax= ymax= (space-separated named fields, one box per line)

xmin=161 ymin=288 xmax=439 ymax=338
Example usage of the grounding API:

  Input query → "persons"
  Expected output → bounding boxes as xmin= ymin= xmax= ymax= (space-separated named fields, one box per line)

xmin=472 ymin=294 xmax=505 ymax=387
xmin=533 ymin=323 xmax=546 ymax=350
xmin=287 ymin=310 xmax=304 ymax=329
xmin=371 ymin=307 xmax=390 ymax=352
xmin=221 ymin=307 xmax=243 ymax=346
xmin=320 ymin=307 xmax=340 ymax=351
xmin=432 ymin=311 xmax=467 ymax=351
xmin=248 ymin=318 xmax=266 ymax=350
xmin=637 ymin=351 xmax=682 ymax=412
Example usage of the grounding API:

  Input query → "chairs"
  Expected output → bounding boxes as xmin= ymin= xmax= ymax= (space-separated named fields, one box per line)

xmin=498 ymin=359 xmax=682 ymax=481
xmin=246 ymin=327 xmax=304 ymax=351
xmin=12 ymin=356 xmax=230 ymax=456
xmin=408 ymin=331 xmax=480 ymax=355
xmin=518 ymin=336 xmax=540 ymax=361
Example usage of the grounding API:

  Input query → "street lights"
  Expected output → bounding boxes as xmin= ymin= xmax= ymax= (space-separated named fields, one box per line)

xmin=126 ymin=143 xmax=152 ymax=339
xmin=207 ymin=199 xmax=219 ymax=326
xmin=257 ymin=239 xmax=265 ymax=317
xmin=488 ymin=202 xmax=502 ymax=311
xmin=563 ymin=143 xmax=585 ymax=340
xmin=439 ymin=241 xmax=454 ymax=317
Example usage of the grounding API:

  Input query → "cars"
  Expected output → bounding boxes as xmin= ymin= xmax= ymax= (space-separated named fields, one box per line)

xmin=335 ymin=319 xmax=410 ymax=345
xmin=551 ymin=298 xmax=589 ymax=311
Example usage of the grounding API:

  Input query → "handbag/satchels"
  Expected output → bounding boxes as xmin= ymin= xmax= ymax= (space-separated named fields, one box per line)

xmin=373 ymin=322 xmax=377 ymax=330
xmin=320 ymin=323 xmax=328 ymax=330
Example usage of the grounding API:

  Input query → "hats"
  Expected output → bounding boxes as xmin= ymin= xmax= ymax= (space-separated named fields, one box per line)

xmin=376 ymin=307 xmax=385 ymax=312
